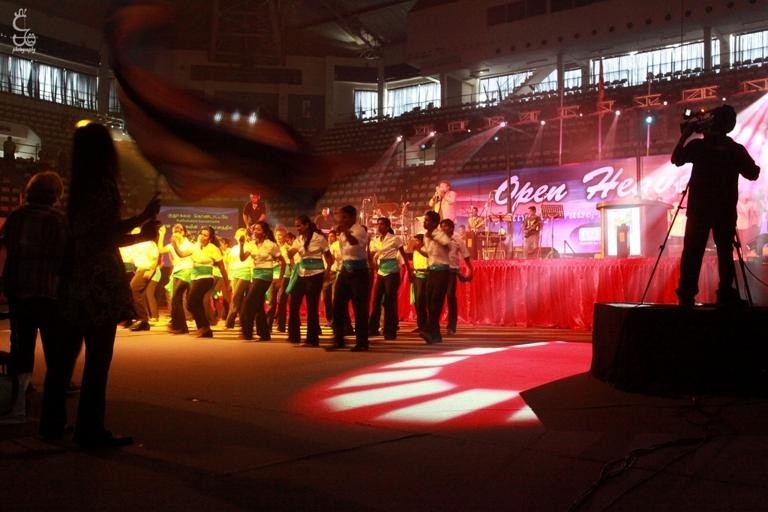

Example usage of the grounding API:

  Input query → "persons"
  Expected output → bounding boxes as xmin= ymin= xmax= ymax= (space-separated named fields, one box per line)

xmin=0 ymin=169 xmax=80 ymax=426
xmin=522 ymin=206 xmax=542 ymax=260
xmin=119 ymin=181 xmax=473 ymax=351
xmin=3 ymin=135 xmax=17 ymax=160
xmin=669 ymin=103 xmax=760 ymax=307
xmin=34 ymin=121 xmax=160 ymax=449
xmin=468 ymin=205 xmax=484 ymax=260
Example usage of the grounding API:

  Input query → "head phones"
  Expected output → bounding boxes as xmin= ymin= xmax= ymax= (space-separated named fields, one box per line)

xmin=711 ymin=105 xmax=732 ymax=128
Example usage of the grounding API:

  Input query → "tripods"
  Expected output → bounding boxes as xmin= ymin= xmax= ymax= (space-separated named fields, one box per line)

xmin=544 ymin=217 xmax=560 ymax=260
xmin=495 ymin=222 xmax=507 ymax=259
xmin=638 ymin=172 xmax=753 ymax=305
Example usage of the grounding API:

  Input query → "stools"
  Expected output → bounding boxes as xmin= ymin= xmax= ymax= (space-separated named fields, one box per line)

xmin=482 ymin=247 xmax=506 ymax=260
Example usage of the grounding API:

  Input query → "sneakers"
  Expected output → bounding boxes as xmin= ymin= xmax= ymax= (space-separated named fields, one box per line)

xmin=79 ymin=430 xmax=134 ymax=453
xmin=325 ymin=341 xmax=345 ymax=351
xmin=1 ymin=410 xmax=27 ymax=425
xmin=121 ymin=318 xmax=151 ymax=332
xmin=297 ymin=341 xmax=320 ymax=348
xmin=412 ymin=327 xmax=443 ymax=345
xmin=446 ymin=328 xmax=454 ymax=336
xmin=225 ymin=310 xmax=401 ymax=341
xmin=349 ymin=344 xmax=369 ymax=352
xmin=194 ymin=328 xmax=214 ymax=339
xmin=166 ymin=320 xmax=189 ymax=334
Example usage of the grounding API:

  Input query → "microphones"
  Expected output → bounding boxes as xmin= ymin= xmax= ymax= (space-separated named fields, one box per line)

xmin=363 ymin=199 xmax=371 ymax=202
xmin=436 ymin=189 xmax=442 ymax=194
xmin=404 ymin=202 xmax=410 ymax=207
